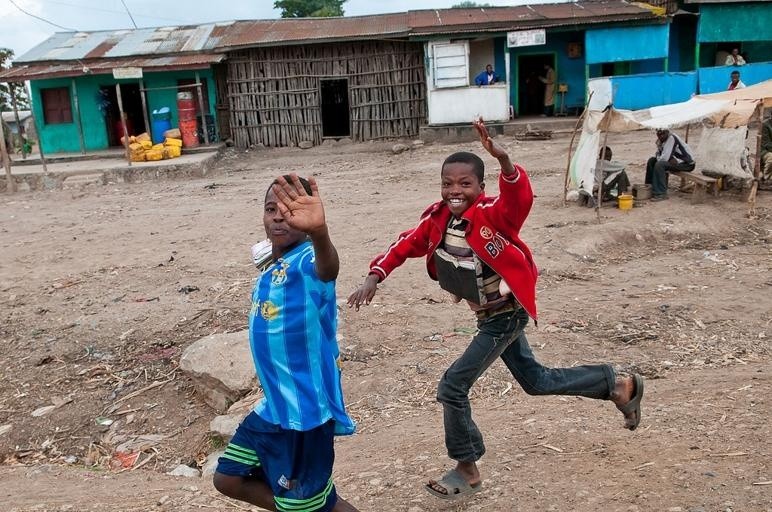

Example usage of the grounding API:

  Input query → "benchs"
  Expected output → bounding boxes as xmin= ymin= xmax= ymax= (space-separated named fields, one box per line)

xmin=669 ymin=170 xmax=716 ymax=204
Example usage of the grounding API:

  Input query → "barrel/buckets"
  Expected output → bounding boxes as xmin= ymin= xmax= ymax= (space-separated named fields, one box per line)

xmin=618 ymin=194 xmax=634 ymax=211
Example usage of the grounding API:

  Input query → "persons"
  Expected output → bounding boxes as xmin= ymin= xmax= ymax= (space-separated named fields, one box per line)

xmin=725 ymin=47 xmax=747 ymax=66
xmin=346 ymin=115 xmax=645 ymax=501
xmin=536 ymin=62 xmax=556 ymax=118
xmin=645 ymin=126 xmax=696 ymax=203
xmin=211 ymin=170 xmax=364 ymax=512
xmin=757 ymin=109 xmax=771 ymax=187
xmin=727 ymin=70 xmax=747 ymax=92
xmin=591 ymin=142 xmax=631 ymax=202
xmin=474 ymin=63 xmax=500 ymax=89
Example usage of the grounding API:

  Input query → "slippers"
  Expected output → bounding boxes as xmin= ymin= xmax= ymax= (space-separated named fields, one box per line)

xmin=616 ymin=374 xmax=644 ymax=431
xmin=423 ymin=470 xmax=486 ymax=501
